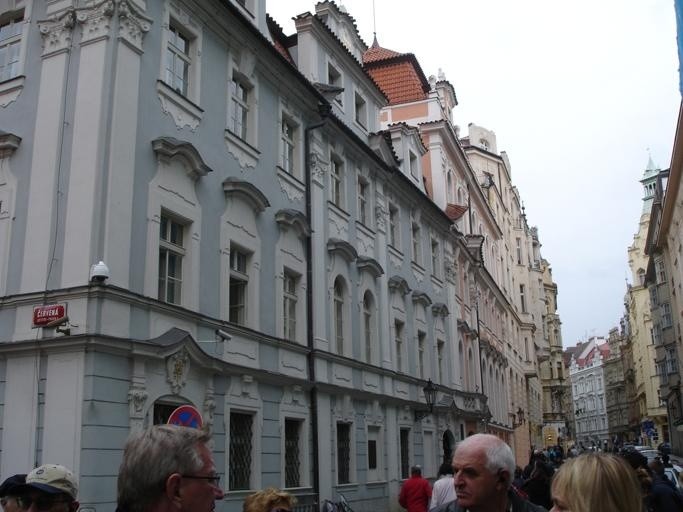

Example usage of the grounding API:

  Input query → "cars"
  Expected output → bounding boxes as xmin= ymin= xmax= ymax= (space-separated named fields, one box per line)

xmin=634 ymin=445 xmax=681 ymax=490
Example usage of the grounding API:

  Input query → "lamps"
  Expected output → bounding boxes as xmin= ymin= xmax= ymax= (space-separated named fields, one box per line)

xmin=87 ymin=260 xmax=109 ymax=288
xmin=423 ymin=377 xmax=437 ymax=413
xmin=516 ymin=406 xmax=526 ymax=427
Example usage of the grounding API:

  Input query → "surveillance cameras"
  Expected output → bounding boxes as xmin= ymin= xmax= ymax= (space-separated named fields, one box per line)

xmin=215 ymin=329 xmax=232 ymax=340
xmin=92 ymin=264 xmax=110 ymax=282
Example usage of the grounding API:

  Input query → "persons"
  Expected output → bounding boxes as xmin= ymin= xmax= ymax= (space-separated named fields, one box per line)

xmin=396 ymin=432 xmax=683 ymax=511
xmin=0 ymin=473 xmax=33 ymax=512
xmin=111 ymin=422 xmax=225 ymax=510
xmin=241 ymin=485 xmax=297 ymax=510
xmin=18 ymin=463 xmax=80 ymax=512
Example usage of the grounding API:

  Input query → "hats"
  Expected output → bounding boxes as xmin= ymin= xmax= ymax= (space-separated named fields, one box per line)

xmin=1 ymin=473 xmax=29 ymax=496
xmin=12 ymin=465 xmax=79 ymax=502
